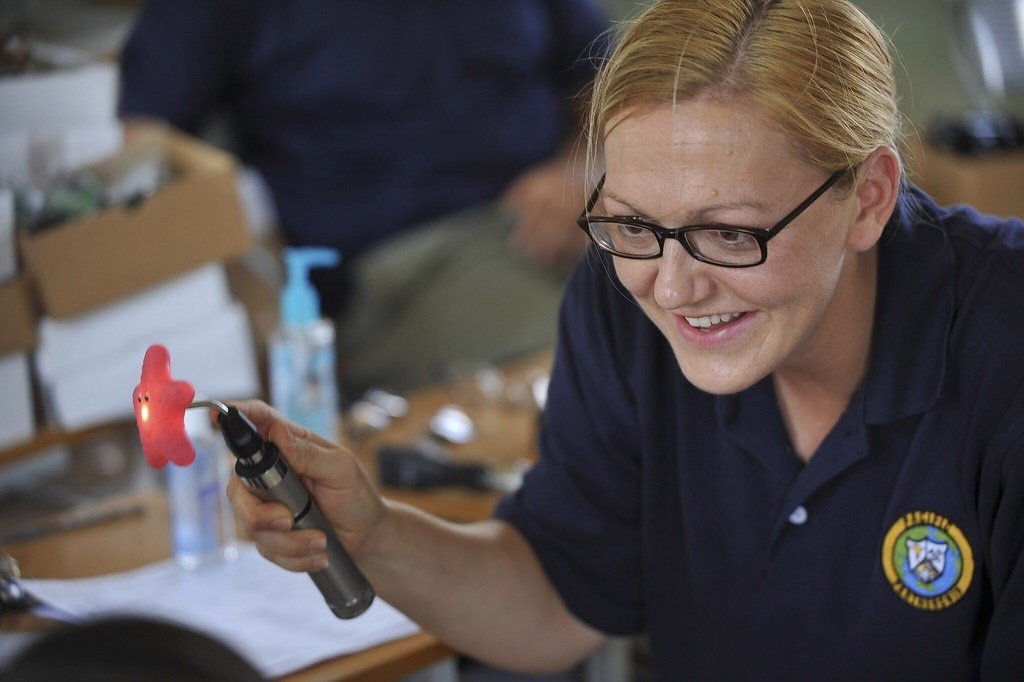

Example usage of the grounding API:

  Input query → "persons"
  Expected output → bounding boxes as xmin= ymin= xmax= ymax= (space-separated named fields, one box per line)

xmin=209 ymin=0 xmax=1024 ymax=682
xmin=118 ymin=0 xmax=624 ymax=323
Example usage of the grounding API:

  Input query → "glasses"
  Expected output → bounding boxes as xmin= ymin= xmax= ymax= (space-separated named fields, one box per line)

xmin=576 ymin=161 xmax=852 ymax=269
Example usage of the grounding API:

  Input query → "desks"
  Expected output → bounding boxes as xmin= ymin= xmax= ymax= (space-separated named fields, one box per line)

xmin=0 ymin=347 xmax=557 ymax=682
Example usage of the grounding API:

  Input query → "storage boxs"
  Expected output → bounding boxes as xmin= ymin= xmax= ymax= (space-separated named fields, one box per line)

xmin=0 ymin=0 xmax=287 ymax=441
